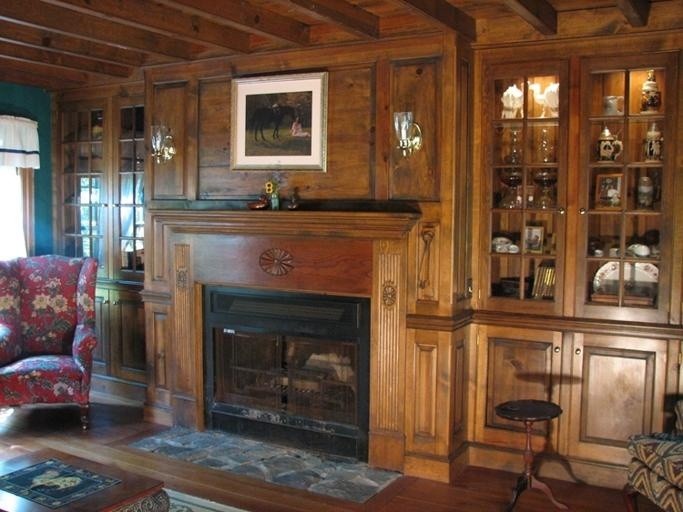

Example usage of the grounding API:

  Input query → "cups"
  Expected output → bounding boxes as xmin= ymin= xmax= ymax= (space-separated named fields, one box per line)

xmin=600 ymin=93 xmax=624 ymax=116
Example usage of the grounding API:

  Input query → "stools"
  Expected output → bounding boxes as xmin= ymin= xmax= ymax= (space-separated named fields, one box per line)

xmin=492 ymin=399 xmax=571 ymax=511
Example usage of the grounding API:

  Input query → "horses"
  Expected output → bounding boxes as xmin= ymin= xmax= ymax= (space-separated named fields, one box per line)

xmin=247 ymin=106 xmax=296 ymax=142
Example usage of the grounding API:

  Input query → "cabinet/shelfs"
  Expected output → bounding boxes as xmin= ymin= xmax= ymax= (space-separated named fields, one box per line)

xmin=49 ymin=77 xmax=145 ymax=402
xmin=469 ymin=29 xmax=682 ymax=491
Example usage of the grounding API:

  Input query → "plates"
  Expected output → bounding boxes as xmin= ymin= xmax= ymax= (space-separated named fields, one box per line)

xmin=592 ymin=260 xmax=659 ymax=298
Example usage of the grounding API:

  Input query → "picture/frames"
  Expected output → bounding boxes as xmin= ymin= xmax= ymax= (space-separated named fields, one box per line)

xmin=592 ymin=170 xmax=624 ymax=211
xmin=521 ymin=224 xmax=544 ymax=255
xmin=229 ymin=71 xmax=328 ymax=172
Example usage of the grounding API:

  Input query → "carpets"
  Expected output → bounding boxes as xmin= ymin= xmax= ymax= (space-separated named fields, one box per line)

xmin=160 ymin=487 xmax=251 ymax=511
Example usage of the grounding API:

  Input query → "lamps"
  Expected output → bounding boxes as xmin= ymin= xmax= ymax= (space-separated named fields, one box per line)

xmin=149 ymin=125 xmax=175 ymax=164
xmin=393 ymin=111 xmax=422 ymax=156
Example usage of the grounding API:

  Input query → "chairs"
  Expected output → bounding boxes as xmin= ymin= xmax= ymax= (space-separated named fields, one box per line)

xmin=0 ymin=256 xmax=97 ymax=432
xmin=617 ymin=394 xmax=683 ymax=511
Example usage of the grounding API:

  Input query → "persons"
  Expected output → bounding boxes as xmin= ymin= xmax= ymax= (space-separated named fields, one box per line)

xmin=291 ymin=115 xmax=311 ymax=138
